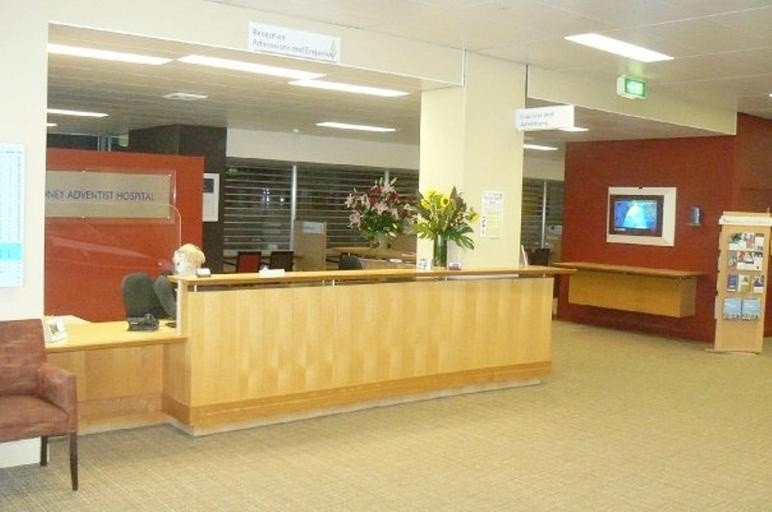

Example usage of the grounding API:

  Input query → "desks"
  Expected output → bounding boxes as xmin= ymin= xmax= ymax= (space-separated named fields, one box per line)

xmin=553 ymin=252 xmax=708 ymax=339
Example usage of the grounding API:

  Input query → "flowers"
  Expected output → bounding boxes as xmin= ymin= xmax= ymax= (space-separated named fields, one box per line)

xmin=341 ymin=169 xmax=414 ymax=238
xmin=406 ymin=185 xmax=479 ymax=252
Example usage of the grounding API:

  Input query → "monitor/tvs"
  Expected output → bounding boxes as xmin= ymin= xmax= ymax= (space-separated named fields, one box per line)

xmin=608 ymin=194 xmax=663 ymax=237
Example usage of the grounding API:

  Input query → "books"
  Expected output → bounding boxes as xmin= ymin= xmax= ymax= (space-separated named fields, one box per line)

xmin=722 ymin=232 xmax=765 ymax=320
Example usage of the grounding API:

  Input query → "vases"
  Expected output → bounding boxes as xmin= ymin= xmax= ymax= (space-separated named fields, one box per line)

xmin=432 ymin=232 xmax=446 ymax=270
xmin=373 ymin=231 xmax=389 ymax=250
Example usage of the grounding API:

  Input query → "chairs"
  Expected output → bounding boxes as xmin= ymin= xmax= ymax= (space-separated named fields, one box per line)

xmin=119 ymin=269 xmax=175 ymax=321
xmin=532 ymin=248 xmax=550 ymax=265
xmin=221 ymin=249 xmax=261 ymax=273
xmin=338 ymin=255 xmax=362 ymax=272
xmin=0 ymin=317 xmax=78 ymax=492
xmin=261 ymin=248 xmax=293 ymax=273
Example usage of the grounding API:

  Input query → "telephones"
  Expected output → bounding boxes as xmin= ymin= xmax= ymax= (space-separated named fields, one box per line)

xmin=126 ymin=313 xmax=159 ymax=331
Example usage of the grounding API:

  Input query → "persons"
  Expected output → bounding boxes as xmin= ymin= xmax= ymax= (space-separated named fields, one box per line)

xmin=151 ymin=243 xmax=206 ymax=319
